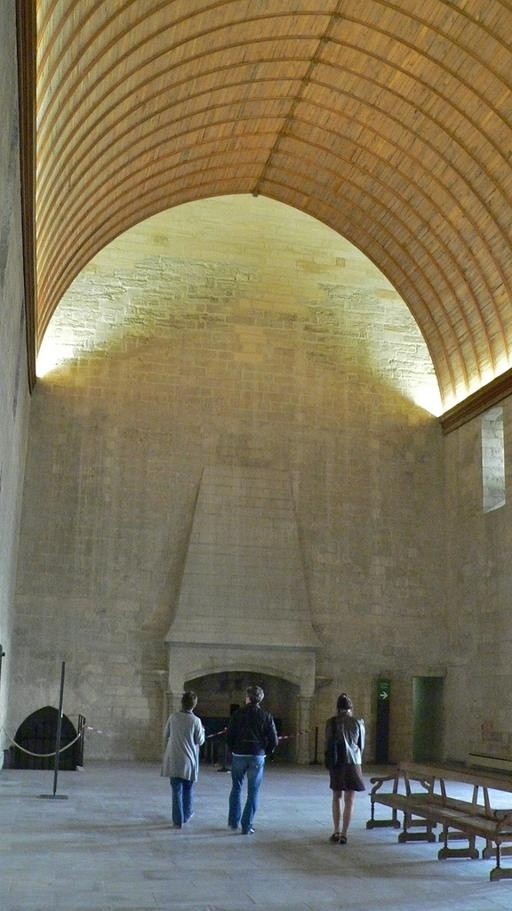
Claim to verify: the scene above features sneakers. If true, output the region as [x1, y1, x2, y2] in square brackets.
[217, 766, 231, 773]
[245, 827, 256, 834]
[331, 832, 347, 844]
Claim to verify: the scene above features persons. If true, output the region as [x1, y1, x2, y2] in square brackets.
[217, 702, 242, 776]
[154, 688, 208, 829]
[323, 691, 367, 844]
[225, 683, 281, 836]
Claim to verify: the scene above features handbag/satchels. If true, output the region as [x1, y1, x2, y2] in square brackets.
[324, 744, 338, 771]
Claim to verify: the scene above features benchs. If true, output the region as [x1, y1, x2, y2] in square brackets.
[366, 762, 512, 882]
